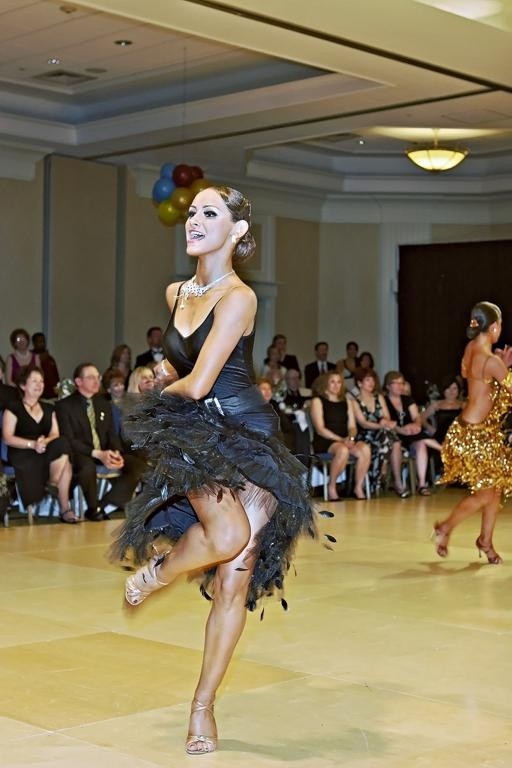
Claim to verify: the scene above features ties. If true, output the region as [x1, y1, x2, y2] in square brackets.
[87, 398, 101, 450]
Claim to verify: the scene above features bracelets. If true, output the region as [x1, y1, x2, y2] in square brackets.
[156, 356, 173, 383]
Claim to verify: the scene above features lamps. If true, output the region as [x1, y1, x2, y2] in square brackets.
[404, 129, 471, 173]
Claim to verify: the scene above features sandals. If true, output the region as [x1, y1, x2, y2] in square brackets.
[125, 550, 177, 605]
[395, 488, 411, 498]
[416, 486, 430, 496]
[58, 509, 81, 523]
[184, 697, 219, 756]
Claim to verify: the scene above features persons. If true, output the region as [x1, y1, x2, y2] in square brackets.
[256, 334, 466, 502]
[3, 365, 83, 524]
[118, 179, 317, 758]
[1, 327, 61, 409]
[102, 326, 181, 511]
[430, 298, 512, 568]
[53, 362, 139, 522]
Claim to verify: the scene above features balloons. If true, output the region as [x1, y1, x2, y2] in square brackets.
[152, 156, 212, 226]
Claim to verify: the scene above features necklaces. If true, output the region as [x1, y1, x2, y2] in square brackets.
[173, 269, 239, 310]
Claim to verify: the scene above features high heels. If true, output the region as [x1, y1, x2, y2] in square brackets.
[428, 521, 451, 558]
[475, 534, 504, 565]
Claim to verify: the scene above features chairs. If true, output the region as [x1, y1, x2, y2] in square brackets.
[2, 411, 443, 523]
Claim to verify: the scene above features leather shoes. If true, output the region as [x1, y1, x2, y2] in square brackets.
[85, 508, 107, 520]
